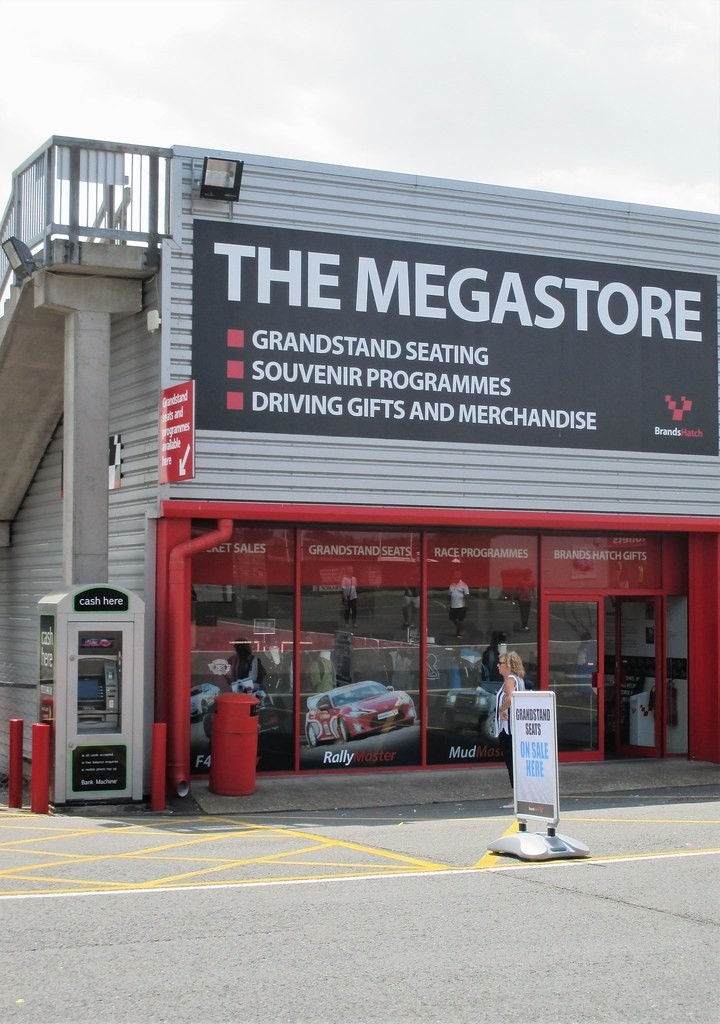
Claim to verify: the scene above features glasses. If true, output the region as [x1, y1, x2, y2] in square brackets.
[498, 661, 506, 665]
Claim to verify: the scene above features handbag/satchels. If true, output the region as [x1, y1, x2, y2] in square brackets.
[342, 594, 351, 606]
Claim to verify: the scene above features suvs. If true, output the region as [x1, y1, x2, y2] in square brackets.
[442, 680, 535, 739]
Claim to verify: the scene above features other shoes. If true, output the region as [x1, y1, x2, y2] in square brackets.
[352, 623, 358, 628]
[344, 624, 349, 628]
[401, 622, 409, 629]
[500, 802, 515, 808]
[410, 623, 415, 629]
[456, 632, 463, 638]
[522, 625, 529, 632]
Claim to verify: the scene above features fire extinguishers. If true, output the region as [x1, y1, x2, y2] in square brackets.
[648, 678, 678, 727]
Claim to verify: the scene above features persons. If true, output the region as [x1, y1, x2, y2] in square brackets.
[401, 562, 419, 630]
[248, 642, 336, 693]
[483, 631, 506, 682]
[447, 570, 470, 638]
[224, 638, 266, 691]
[515, 569, 534, 632]
[342, 565, 377, 628]
[495, 652, 525, 809]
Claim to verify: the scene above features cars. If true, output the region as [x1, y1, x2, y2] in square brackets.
[306, 680, 416, 746]
[189, 683, 220, 719]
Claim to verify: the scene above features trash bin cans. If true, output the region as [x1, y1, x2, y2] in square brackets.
[208, 692, 260, 797]
